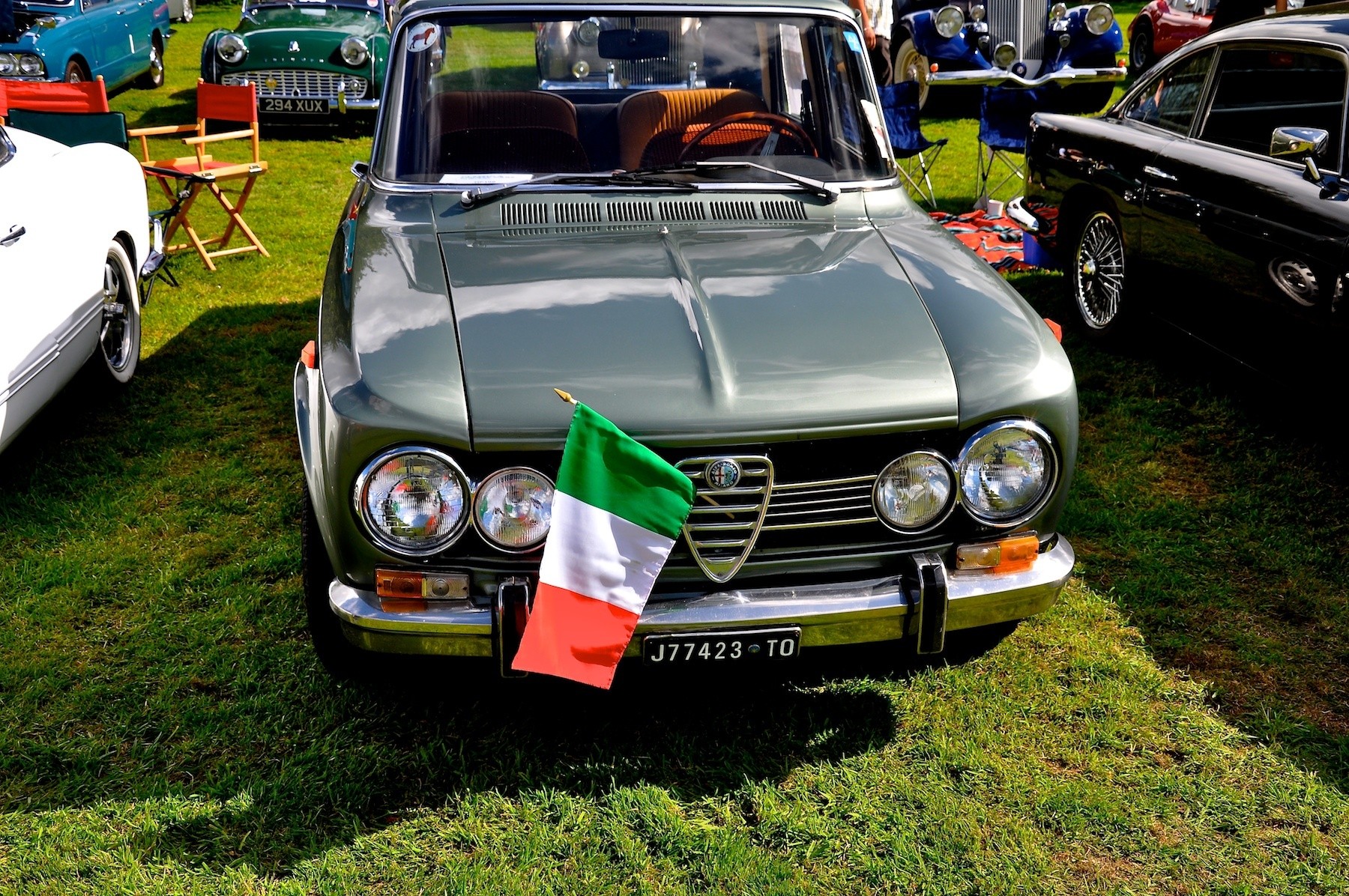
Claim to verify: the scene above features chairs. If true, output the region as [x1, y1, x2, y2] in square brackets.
[425, 89, 580, 165]
[7, 106, 187, 309]
[0, 75, 112, 128]
[973, 82, 1049, 208]
[127, 77, 272, 272]
[876, 78, 950, 210]
[616, 84, 769, 169]
[428, 126, 592, 173]
[637, 120, 805, 167]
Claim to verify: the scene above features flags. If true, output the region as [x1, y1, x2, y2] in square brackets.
[513, 398, 696, 693]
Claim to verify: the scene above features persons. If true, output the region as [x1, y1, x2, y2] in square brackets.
[1027, 122, 1056, 190]
[1020, 466, 1034, 489]
[848, 0, 895, 89]
[1128, 78, 1167, 126]
[1208, 0, 1288, 38]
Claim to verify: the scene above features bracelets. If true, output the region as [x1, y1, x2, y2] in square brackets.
[863, 26, 871, 31]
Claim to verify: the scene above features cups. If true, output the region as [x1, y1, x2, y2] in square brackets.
[987, 198, 1005, 216]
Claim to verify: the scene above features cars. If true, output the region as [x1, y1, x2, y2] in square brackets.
[201, 1, 392, 137]
[1, 125, 150, 454]
[292, 0, 1082, 669]
[0, 0, 170, 89]
[1023, 15, 1348, 414]
